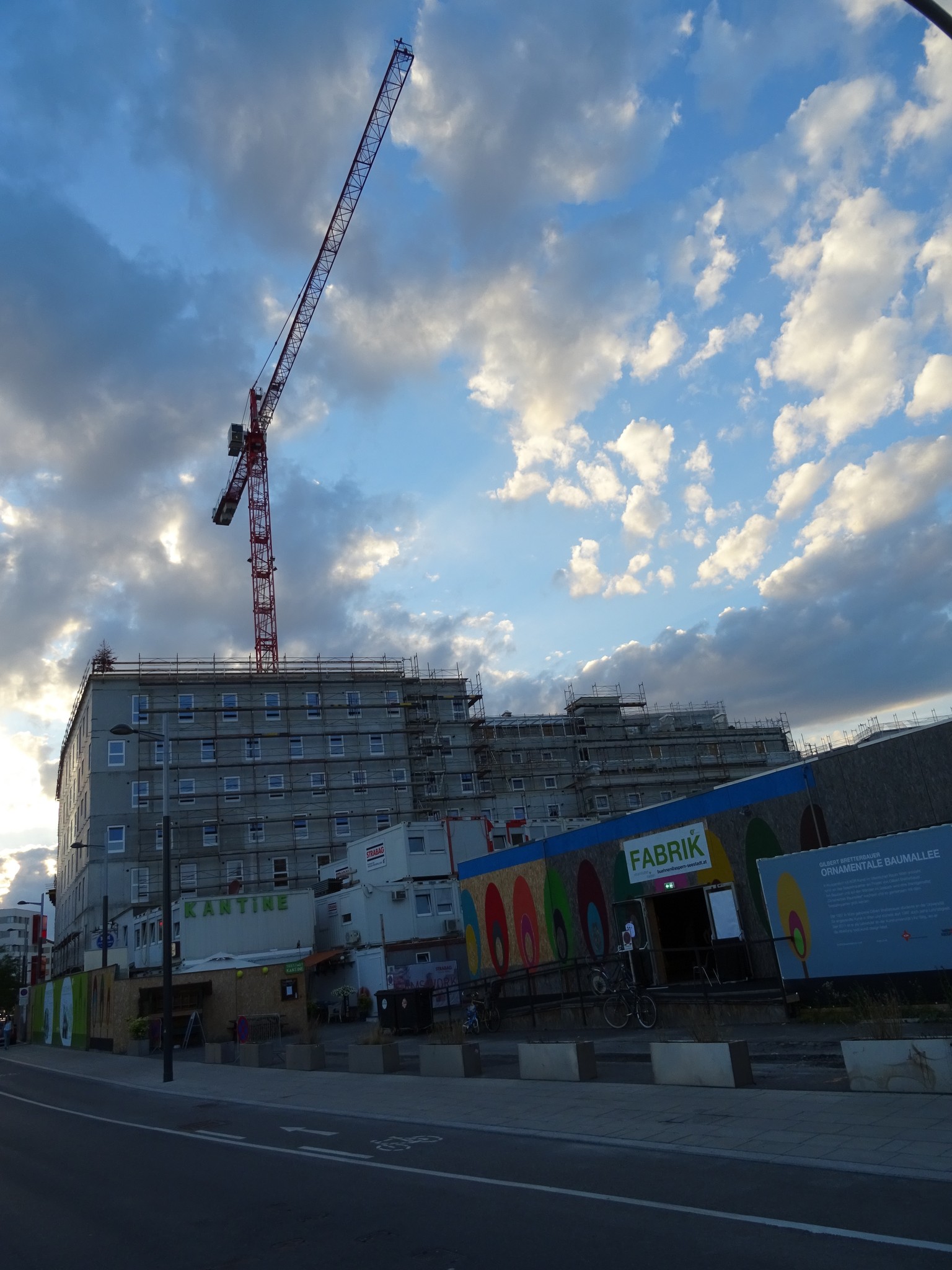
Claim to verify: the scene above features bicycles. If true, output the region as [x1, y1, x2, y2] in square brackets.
[586, 947, 637, 996]
[461, 991, 500, 1033]
[603, 978, 657, 1029]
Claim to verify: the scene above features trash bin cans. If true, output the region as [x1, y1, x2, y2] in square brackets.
[374, 985, 437, 1037]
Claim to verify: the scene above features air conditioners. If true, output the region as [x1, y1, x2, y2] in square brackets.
[345, 930, 359, 944]
[444, 919, 460, 932]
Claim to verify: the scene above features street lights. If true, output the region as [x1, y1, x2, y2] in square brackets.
[108, 713, 172, 1080]
[6, 918, 28, 985]
[70, 830, 108, 967]
[17, 893, 44, 984]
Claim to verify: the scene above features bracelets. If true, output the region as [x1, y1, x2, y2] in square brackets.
[12, 1030, 14, 1030]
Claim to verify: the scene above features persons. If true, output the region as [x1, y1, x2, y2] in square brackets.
[1, 1015, 14, 1051]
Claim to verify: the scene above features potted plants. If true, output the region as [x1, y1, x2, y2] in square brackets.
[124, 986, 952, 1094]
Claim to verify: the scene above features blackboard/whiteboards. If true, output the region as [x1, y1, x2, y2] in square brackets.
[185, 1011, 205, 1044]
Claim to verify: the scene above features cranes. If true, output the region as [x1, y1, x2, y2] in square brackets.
[212, 36, 418, 673]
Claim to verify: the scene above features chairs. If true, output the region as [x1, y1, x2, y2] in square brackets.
[328, 1000, 344, 1024]
